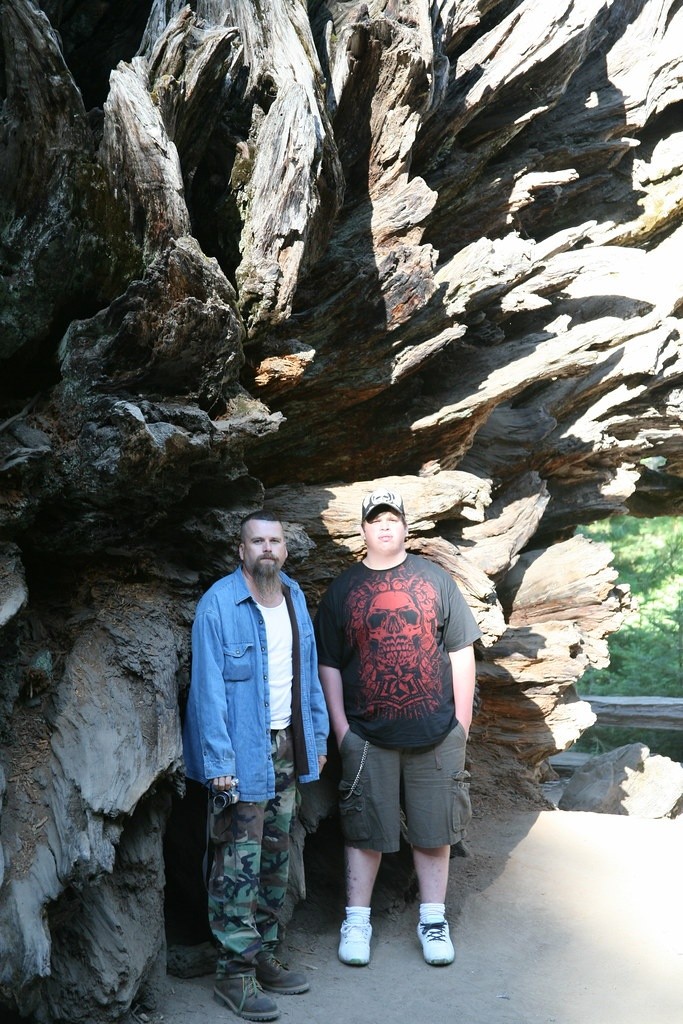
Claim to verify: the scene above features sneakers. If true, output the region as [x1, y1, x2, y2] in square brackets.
[417, 920, 456, 965]
[337, 920, 373, 967]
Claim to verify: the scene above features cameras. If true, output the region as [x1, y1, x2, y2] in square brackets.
[213, 778, 240, 809]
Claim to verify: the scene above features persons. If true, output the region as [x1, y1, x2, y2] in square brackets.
[314, 488, 482, 965]
[184, 510, 329, 1021]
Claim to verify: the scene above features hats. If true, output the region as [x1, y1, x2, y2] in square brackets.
[360, 491, 405, 524]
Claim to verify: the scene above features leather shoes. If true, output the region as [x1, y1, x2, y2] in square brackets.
[214, 975, 280, 1021]
[256, 947, 309, 995]
